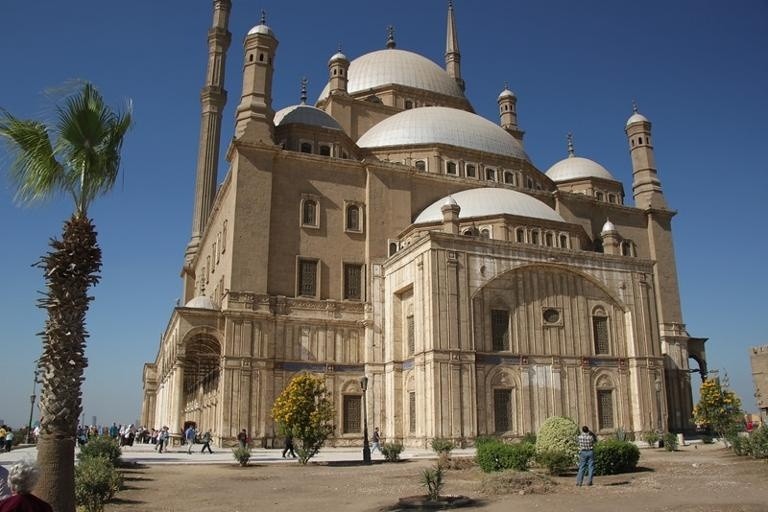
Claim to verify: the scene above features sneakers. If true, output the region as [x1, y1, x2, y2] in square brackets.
[120, 442, 215, 454]
[283, 455, 297, 459]
[576, 482, 593, 486]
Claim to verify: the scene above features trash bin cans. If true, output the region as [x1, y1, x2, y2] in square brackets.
[677, 433, 684, 446]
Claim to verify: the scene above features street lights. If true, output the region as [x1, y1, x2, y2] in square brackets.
[359, 377, 373, 463]
[25, 393, 37, 444]
[81, 412, 85, 427]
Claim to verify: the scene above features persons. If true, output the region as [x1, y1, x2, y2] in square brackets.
[371, 427, 382, 454]
[0, 460, 53, 512]
[0, 422, 253, 454]
[575, 426, 595, 486]
[0, 465, 11, 499]
[282, 432, 296, 458]
